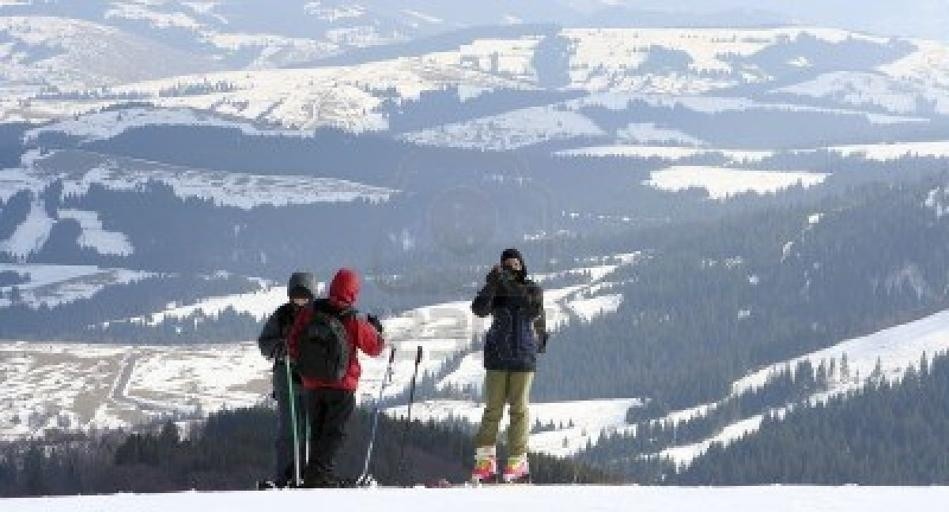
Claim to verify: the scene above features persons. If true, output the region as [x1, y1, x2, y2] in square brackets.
[466, 247, 549, 483]
[287, 266, 385, 488]
[254, 272, 320, 488]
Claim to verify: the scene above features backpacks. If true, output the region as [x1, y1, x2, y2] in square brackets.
[294, 299, 361, 384]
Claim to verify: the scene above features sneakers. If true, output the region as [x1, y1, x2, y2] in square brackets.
[499, 453, 531, 482]
[470, 455, 497, 481]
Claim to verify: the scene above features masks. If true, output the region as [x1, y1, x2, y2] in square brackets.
[293, 298, 310, 307]
